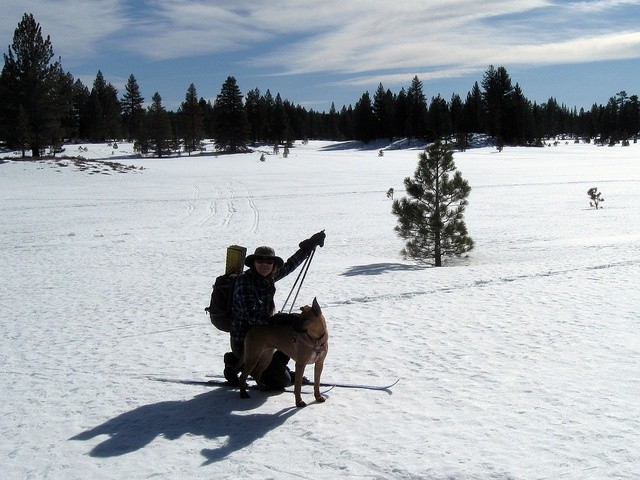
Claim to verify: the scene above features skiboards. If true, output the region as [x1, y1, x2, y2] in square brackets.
[147, 375, 398, 396]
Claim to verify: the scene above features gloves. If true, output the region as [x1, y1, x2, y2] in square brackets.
[298, 231, 325, 253]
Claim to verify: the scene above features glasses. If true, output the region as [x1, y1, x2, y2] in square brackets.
[256, 257, 274, 264]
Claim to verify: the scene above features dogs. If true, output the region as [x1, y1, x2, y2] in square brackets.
[239, 296, 329, 408]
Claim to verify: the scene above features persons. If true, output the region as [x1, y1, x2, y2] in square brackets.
[223, 231, 328, 388]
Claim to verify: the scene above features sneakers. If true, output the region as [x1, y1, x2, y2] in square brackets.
[290, 371, 309, 383]
[224, 352, 240, 381]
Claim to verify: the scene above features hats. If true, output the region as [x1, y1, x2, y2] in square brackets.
[244, 246, 285, 269]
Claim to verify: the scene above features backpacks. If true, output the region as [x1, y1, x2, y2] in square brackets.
[205, 272, 239, 332]
